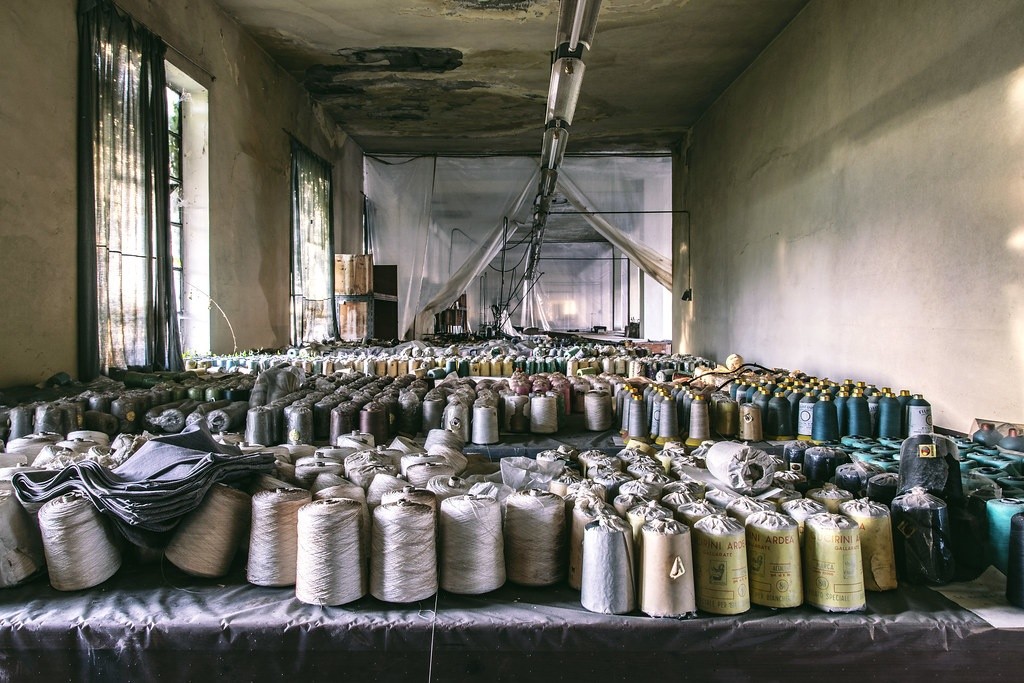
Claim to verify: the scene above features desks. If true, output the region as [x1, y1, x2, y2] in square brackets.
[0, 558, 1024, 683]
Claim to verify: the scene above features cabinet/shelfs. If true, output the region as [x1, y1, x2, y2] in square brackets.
[434, 293, 467, 333]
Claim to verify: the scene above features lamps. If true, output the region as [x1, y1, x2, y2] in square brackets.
[538, 126, 568, 169]
[544, 54, 587, 126]
[525, 166, 559, 280]
[554, 0, 603, 52]
